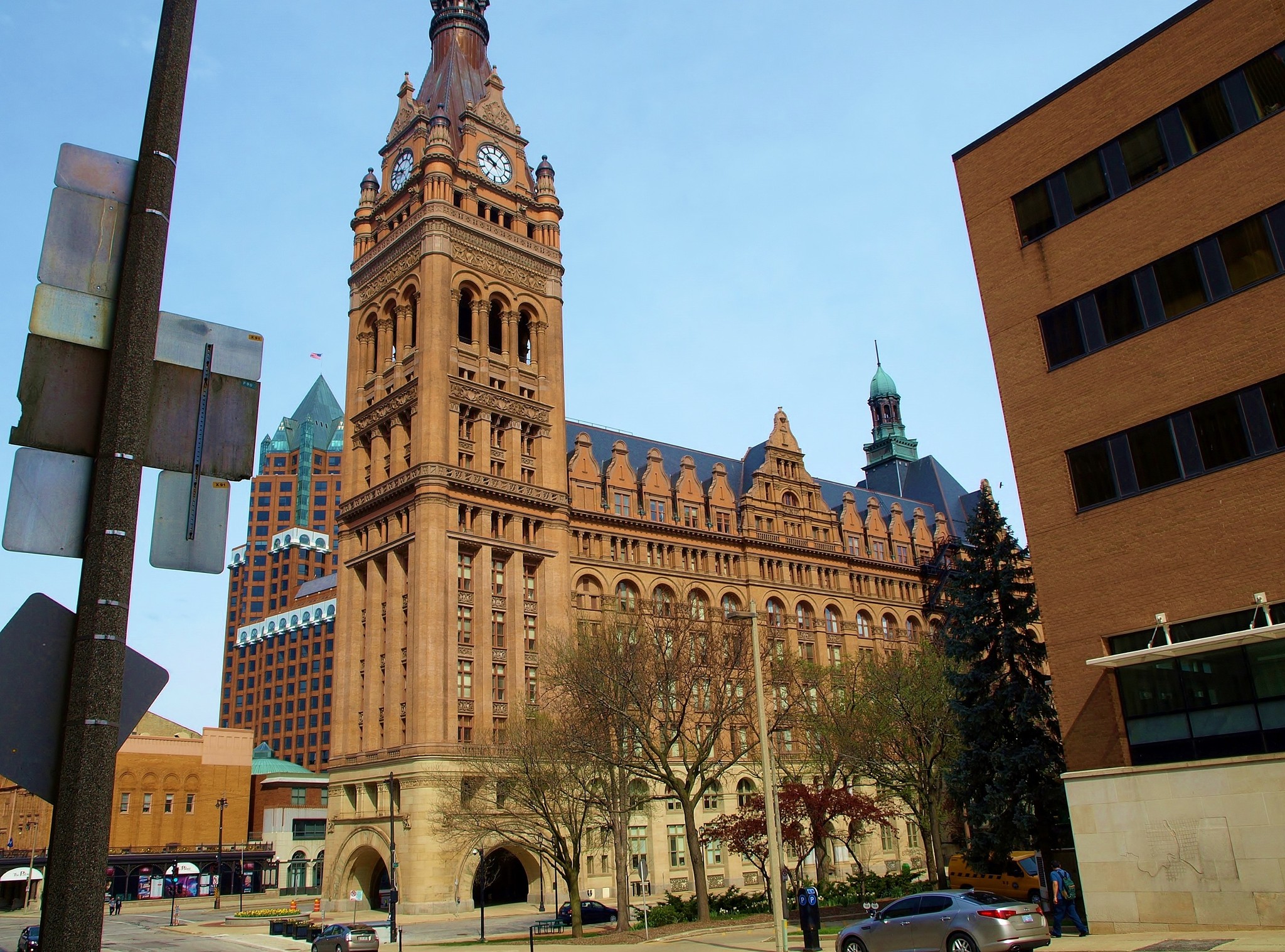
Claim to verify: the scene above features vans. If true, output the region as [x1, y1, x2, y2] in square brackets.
[948, 850, 1042, 906]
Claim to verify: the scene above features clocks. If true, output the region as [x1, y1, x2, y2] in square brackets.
[390, 150, 414, 192]
[476, 143, 513, 185]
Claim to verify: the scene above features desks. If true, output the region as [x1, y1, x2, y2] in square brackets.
[535, 919, 564, 933]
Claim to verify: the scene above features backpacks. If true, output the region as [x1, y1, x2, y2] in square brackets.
[1055, 870, 1076, 900]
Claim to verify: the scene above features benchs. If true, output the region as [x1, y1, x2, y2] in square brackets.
[532, 924, 568, 935]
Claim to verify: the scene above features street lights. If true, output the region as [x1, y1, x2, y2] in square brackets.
[550, 834, 561, 928]
[725, 600, 785, 952]
[537, 832, 547, 912]
[383, 771, 398, 943]
[473, 847, 488, 944]
[276, 856, 280, 888]
[23, 820, 38, 914]
[213, 798, 229, 896]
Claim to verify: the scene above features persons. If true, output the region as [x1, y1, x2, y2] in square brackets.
[242, 875, 252, 893]
[115, 896, 122, 915]
[1046, 860, 1090, 939]
[108, 897, 116, 916]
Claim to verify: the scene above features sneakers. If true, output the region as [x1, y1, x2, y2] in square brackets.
[1049, 930, 1061, 938]
[1079, 931, 1088, 937]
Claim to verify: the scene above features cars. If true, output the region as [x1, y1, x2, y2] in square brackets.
[835, 888, 1052, 952]
[310, 924, 379, 952]
[559, 900, 618, 926]
[17, 925, 40, 952]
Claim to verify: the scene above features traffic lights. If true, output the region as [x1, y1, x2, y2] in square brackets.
[174, 867, 179, 883]
[171, 865, 174, 882]
[178, 883, 183, 894]
